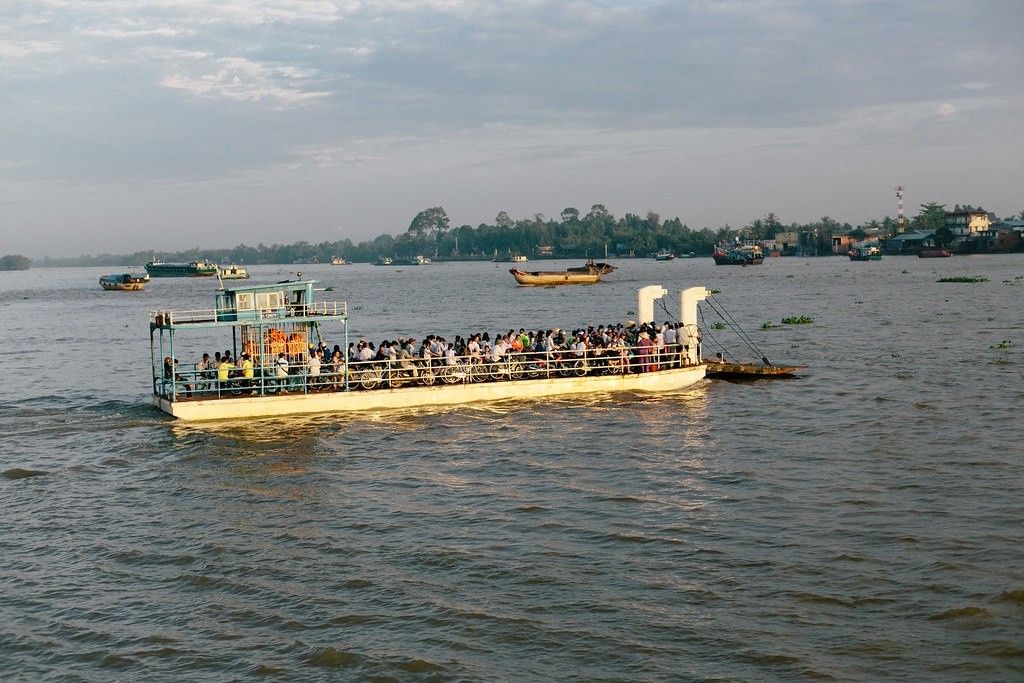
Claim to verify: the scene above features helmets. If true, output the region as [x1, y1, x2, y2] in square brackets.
[554, 328, 561, 334]
[242, 351, 246, 356]
[164, 357, 171, 363]
[321, 342, 327, 347]
[360, 339, 366, 343]
[308, 343, 314, 349]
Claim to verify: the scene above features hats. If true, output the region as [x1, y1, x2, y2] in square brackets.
[398, 337, 403, 341]
[639, 332, 650, 339]
[278, 353, 285, 356]
[503, 335, 508, 340]
[409, 338, 417, 341]
[650, 321, 658, 325]
[626, 320, 635, 328]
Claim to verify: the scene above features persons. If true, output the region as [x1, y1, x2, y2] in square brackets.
[164, 357, 194, 400]
[198, 321, 703, 399]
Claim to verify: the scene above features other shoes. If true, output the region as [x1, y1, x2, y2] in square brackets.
[285, 390, 288, 393]
[309, 390, 311, 392]
[187, 397, 194, 400]
[316, 390, 319, 392]
[221, 396, 223, 398]
[627, 370, 634, 374]
[279, 392, 281, 395]
[176, 393, 181, 396]
[250, 392, 253, 395]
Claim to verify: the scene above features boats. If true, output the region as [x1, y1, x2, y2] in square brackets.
[713, 245, 765, 265]
[144, 260, 215, 277]
[99, 271, 148, 290]
[147, 283, 808, 423]
[656, 252, 673, 261]
[918, 250, 950, 259]
[848, 246, 882, 261]
[510, 262, 618, 286]
[680, 252, 694, 258]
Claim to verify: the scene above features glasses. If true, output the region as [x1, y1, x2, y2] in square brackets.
[520, 332, 523, 334]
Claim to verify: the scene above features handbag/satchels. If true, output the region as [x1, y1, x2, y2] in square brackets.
[650, 363, 657, 372]
